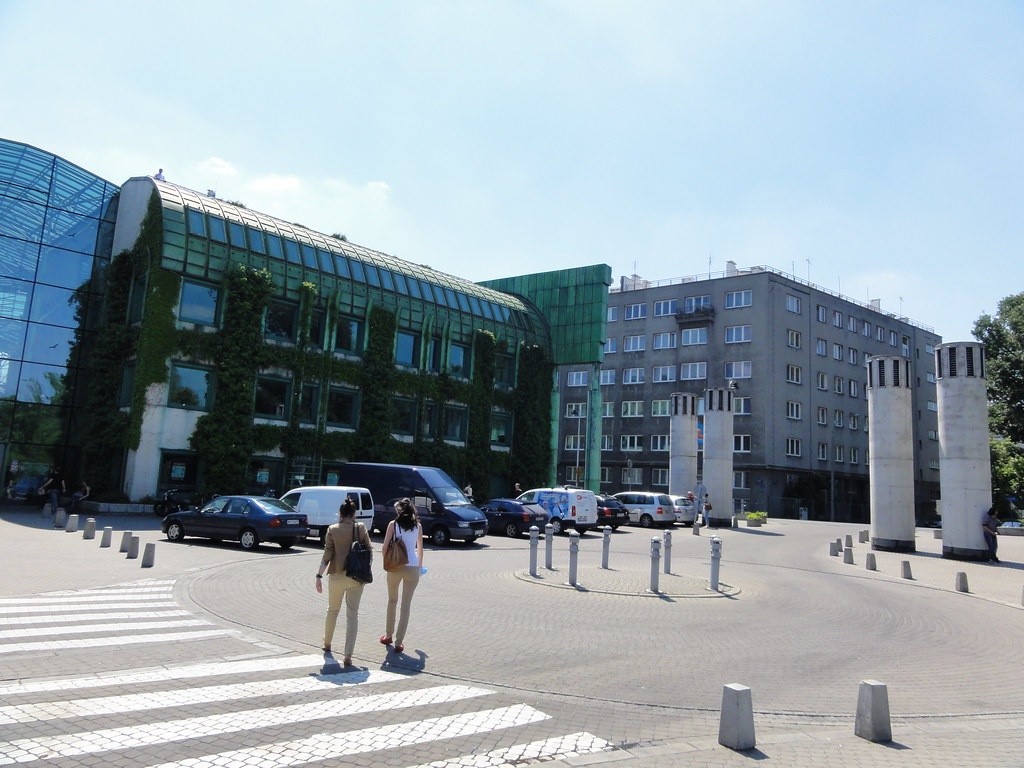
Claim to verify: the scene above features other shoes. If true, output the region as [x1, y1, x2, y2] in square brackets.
[380, 635, 393, 644]
[395, 644, 404, 653]
[322, 645, 331, 651]
[343, 658, 353, 665]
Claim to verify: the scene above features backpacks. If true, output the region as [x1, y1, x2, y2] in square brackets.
[463, 489, 468, 494]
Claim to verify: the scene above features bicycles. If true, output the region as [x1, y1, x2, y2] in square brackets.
[154, 489, 187, 517]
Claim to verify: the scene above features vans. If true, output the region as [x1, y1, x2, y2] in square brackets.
[612, 491, 676, 529]
[514, 488, 598, 536]
[337, 462, 489, 547]
[279, 486, 374, 547]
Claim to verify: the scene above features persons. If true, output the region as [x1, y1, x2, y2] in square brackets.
[463, 482, 472, 504]
[702, 492, 712, 529]
[982, 507, 1003, 563]
[687, 491, 695, 503]
[513, 483, 522, 497]
[316, 497, 373, 665]
[380, 497, 423, 651]
[264, 490, 275, 498]
[68, 480, 92, 514]
[37, 467, 66, 514]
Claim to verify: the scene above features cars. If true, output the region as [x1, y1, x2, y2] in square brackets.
[478, 499, 549, 538]
[588, 494, 630, 531]
[14, 475, 47, 501]
[160, 495, 311, 551]
[667, 495, 699, 526]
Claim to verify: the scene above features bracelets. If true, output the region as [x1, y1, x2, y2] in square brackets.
[42, 485, 45, 488]
[62, 488, 66, 490]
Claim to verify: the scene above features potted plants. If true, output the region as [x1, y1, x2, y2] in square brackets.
[746, 512, 761, 527]
[756, 511, 768, 524]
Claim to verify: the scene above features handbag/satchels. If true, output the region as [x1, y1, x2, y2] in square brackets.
[38, 489, 45, 495]
[383, 521, 409, 572]
[344, 523, 373, 584]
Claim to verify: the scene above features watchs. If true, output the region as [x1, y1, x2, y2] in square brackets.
[316, 573, 323, 578]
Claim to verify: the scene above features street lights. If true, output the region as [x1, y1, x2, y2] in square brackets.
[571, 410, 581, 487]
